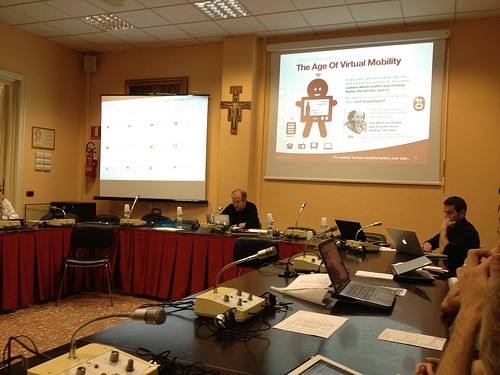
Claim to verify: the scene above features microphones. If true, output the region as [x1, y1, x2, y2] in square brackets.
[284, 202, 309, 238]
[119, 194, 146, 227]
[195, 226, 338, 321]
[47, 206, 75, 226]
[27, 307, 167, 375]
[355, 221, 382, 241]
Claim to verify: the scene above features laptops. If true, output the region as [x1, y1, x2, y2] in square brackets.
[317, 238, 396, 310]
[283, 355, 365, 375]
[392, 256, 435, 283]
[386, 227, 448, 258]
[335, 220, 390, 247]
[206, 215, 230, 226]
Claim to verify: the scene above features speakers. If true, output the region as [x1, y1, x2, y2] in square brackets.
[84, 55, 97, 72]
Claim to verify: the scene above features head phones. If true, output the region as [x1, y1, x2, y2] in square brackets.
[259, 292, 278, 315]
[215, 311, 241, 333]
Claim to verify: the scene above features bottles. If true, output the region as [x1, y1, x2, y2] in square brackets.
[267, 221, 275, 239]
[124, 210, 130, 219]
[177, 214, 183, 229]
[321, 224, 327, 238]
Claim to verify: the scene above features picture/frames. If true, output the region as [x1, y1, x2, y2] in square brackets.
[32, 126, 55, 150]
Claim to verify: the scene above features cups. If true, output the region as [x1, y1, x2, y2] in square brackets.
[321, 217, 327, 226]
[267, 213, 273, 222]
[177, 206, 183, 215]
[124, 204, 130, 212]
[307, 230, 313, 244]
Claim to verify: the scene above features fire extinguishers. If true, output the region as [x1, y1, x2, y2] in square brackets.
[86, 141, 97, 177]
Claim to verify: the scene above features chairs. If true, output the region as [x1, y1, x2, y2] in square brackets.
[54, 222, 116, 308]
[365, 232, 386, 243]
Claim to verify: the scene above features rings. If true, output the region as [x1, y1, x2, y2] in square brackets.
[463, 264, 467, 267]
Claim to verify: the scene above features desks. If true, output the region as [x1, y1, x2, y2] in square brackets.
[0, 219, 440, 375]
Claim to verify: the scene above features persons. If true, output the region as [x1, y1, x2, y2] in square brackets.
[415, 188, 500, 375]
[346, 110, 365, 134]
[0, 186, 19, 221]
[422, 196, 479, 266]
[225, 98, 245, 128]
[221, 189, 260, 229]
